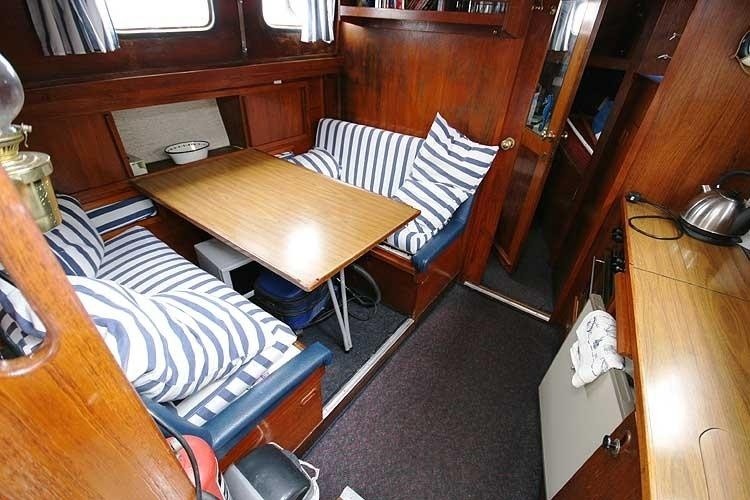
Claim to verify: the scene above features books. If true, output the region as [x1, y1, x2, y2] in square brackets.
[375, 0, 454, 12]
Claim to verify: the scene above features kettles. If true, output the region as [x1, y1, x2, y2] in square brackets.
[678, 169, 750, 246]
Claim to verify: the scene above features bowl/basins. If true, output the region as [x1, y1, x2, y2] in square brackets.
[163, 140, 209, 165]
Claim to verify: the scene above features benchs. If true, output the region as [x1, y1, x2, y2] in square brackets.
[95, 224, 334, 477]
[278, 117, 474, 321]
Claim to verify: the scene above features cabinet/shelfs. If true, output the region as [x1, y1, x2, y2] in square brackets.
[493, 3, 749, 325]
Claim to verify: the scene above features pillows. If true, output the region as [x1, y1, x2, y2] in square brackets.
[286, 146, 343, 180]
[64, 274, 277, 402]
[44, 192, 104, 279]
[380, 114, 499, 254]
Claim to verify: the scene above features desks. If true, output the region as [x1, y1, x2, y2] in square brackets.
[133, 146, 420, 352]
[549, 264, 750, 500]
[555, 195, 746, 298]
